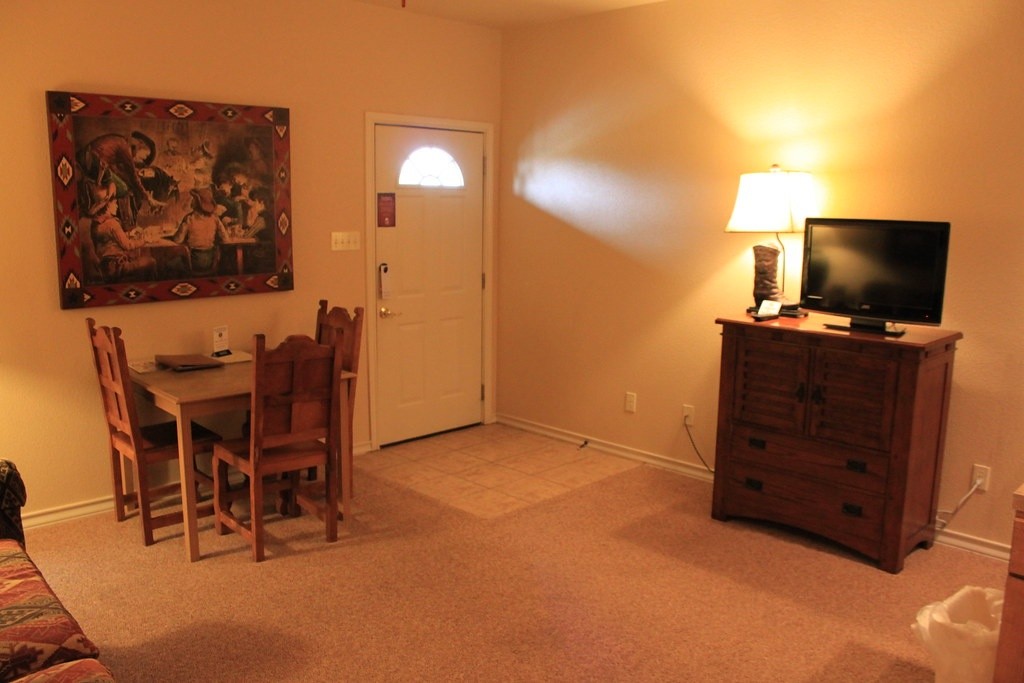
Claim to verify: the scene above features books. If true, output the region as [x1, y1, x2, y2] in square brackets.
[155, 353, 225, 371]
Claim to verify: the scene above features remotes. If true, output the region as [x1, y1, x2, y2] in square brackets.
[752, 313, 779, 322]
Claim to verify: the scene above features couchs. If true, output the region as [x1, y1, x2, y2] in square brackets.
[0, 457, 119, 683]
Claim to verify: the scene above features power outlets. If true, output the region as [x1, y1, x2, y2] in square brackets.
[624, 392, 636, 413]
[682, 404, 695, 427]
[969, 464, 989, 493]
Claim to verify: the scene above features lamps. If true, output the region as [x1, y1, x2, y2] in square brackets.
[723, 164, 832, 318]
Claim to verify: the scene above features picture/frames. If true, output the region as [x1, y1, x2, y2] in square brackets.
[44, 90, 295, 311]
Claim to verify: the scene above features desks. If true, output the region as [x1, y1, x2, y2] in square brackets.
[126, 349, 360, 563]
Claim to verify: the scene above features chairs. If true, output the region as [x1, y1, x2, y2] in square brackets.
[218, 299, 364, 562]
[84, 316, 232, 546]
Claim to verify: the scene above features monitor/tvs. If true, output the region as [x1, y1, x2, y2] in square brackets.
[799, 218, 951, 336]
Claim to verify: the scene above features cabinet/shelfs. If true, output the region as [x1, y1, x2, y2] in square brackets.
[711, 308, 964, 574]
